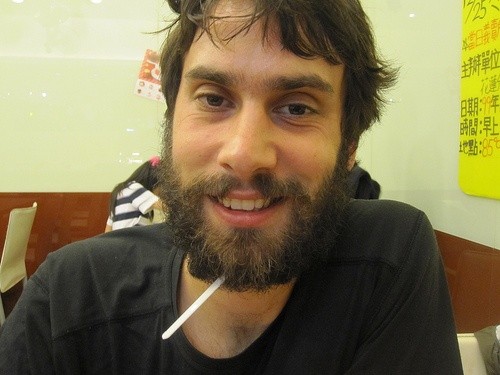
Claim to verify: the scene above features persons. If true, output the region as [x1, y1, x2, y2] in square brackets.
[347, 162, 378, 200]
[105, 156, 166, 232]
[0, 0, 463, 375]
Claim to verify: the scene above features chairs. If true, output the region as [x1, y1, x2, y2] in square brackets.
[0, 201, 38, 327]
